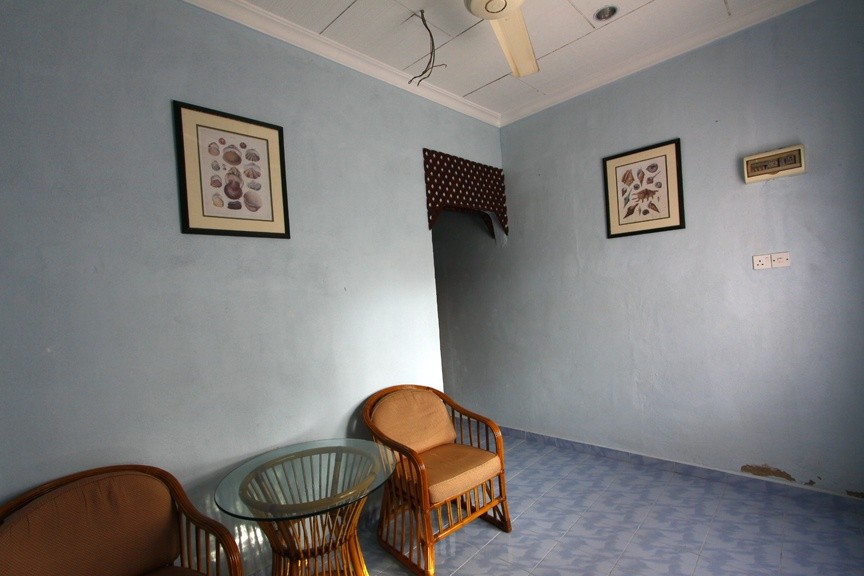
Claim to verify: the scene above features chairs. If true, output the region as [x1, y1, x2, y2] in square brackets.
[0, 464, 244, 576]
[362, 383, 512, 576]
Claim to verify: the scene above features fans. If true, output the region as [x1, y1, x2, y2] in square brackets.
[464, 0, 540, 78]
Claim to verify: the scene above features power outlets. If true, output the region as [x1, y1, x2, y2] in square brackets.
[752, 251, 792, 270]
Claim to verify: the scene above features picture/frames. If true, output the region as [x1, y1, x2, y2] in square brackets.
[601, 136, 686, 240]
[169, 99, 291, 239]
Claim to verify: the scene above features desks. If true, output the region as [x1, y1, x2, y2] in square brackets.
[213, 438, 398, 576]
